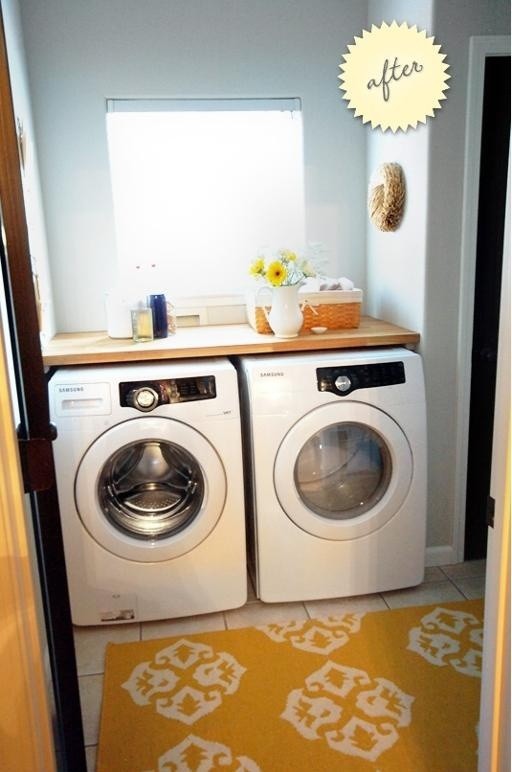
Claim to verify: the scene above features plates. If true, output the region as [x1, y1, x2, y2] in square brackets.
[310, 326, 327, 333]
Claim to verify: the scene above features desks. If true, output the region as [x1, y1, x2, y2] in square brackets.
[38, 311, 424, 369]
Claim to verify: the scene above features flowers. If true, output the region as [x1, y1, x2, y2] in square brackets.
[248, 249, 318, 289]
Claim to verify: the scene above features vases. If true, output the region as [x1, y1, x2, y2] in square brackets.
[255, 281, 310, 342]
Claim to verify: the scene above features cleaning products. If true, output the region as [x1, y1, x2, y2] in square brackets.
[131, 300, 153, 344]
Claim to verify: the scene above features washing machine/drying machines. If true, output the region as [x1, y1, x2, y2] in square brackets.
[46, 356, 249, 630]
[233, 346, 429, 605]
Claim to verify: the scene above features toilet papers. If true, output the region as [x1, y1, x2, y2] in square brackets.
[105, 294, 136, 340]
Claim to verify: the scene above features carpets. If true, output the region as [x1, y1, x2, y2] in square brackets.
[87, 592, 490, 772]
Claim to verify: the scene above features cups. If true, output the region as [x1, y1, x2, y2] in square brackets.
[105, 296, 148, 339]
[132, 310, 154, 342]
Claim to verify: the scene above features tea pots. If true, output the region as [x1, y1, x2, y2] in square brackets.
[257, 281, 307, 338]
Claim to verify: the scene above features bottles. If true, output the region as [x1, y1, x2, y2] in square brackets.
[150, 294, 168, 340]
